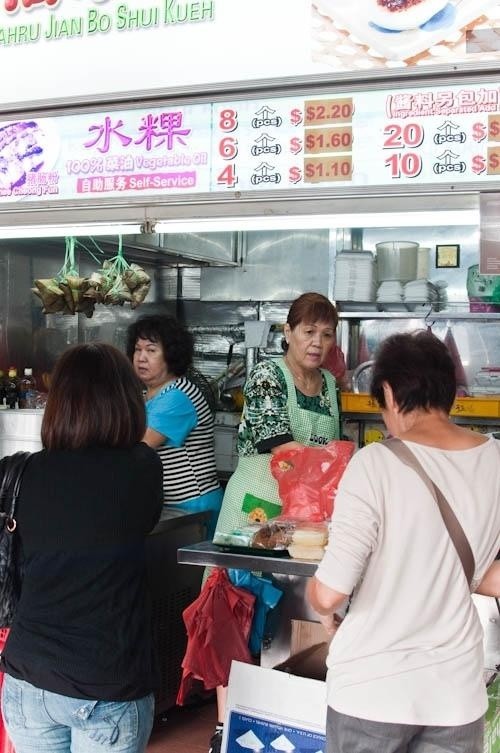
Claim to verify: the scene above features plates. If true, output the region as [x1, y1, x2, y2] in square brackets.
[351, 359, 376, 394]
[375, 280, 440, 303]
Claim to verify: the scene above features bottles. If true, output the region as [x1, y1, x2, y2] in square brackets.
[0, 366, 37, 410]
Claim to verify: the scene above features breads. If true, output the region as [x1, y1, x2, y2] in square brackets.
[212, 518, 328, 560]
[366, 0, 449, 31]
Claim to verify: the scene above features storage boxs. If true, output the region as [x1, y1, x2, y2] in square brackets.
[220, 617, 340, 753]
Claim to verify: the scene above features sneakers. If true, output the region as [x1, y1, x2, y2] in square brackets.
[208, 733, 222, 753]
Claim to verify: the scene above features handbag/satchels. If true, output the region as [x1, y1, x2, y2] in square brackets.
[0, 450, 39, 631]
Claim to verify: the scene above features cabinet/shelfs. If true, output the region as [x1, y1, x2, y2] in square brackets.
[63, 231, 247, 269]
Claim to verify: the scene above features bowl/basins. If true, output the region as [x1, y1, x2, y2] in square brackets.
[376, 241, 419, 281]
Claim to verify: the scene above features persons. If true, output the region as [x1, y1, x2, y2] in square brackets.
[1, 344, 170, 752]
[20, 351, 58, 393]
[305, 325, 500, 752]
[120, 314, 223, 550]
[201, 292, 339, 753]
